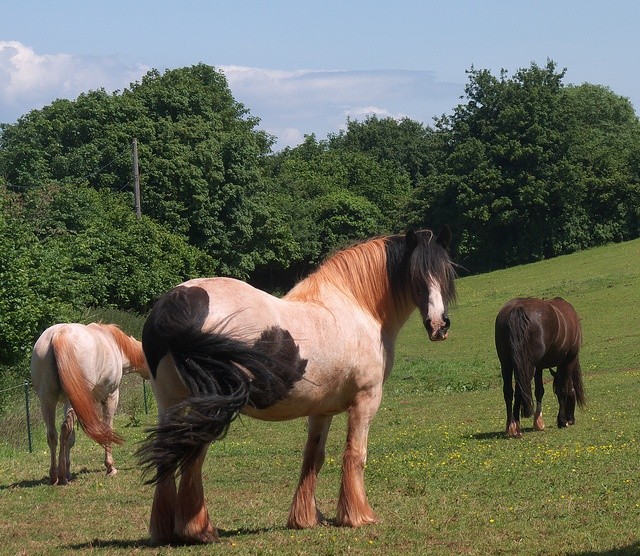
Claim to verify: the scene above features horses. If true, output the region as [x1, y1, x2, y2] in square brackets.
[132, 229, 469, 543]
[497, 297, 588, 438]
[30, 322, 148, 487]
